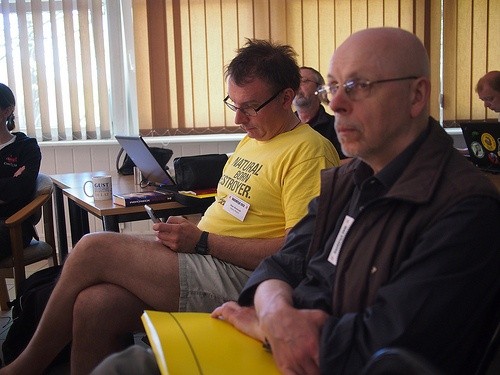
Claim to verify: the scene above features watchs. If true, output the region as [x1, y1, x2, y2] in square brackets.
[196, 232, 209, 255]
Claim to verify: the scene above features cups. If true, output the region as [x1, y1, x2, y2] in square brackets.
[133, 166, 143, 185]
[83, 176, 112, 201]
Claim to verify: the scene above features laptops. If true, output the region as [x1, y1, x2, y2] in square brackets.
[460, 121, 500, 174]
[114, 136, 212, 192]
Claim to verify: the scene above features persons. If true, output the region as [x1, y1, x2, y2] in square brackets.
[476, 70, 500, 122]
[293, 68, 353, 165]
[0, 37, 340, 375]
[92, 27, 500, 375]
[0, 83, 42, 260]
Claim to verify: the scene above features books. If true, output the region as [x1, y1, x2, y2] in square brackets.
[114, 192, 164, 206]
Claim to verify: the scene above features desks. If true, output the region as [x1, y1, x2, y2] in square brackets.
[62, 183, 200, 248]
[43, 171, 135, 264]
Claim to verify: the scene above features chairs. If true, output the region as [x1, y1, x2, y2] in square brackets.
[0, 172, 59, 312]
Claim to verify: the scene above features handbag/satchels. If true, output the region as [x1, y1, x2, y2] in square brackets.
[2, 265, 135, 375]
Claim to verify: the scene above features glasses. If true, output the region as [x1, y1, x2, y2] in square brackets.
[314, 76, 417, 105]
[223, 92, 279, 117]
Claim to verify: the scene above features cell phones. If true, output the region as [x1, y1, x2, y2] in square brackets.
[144, 204, 162, 223]
